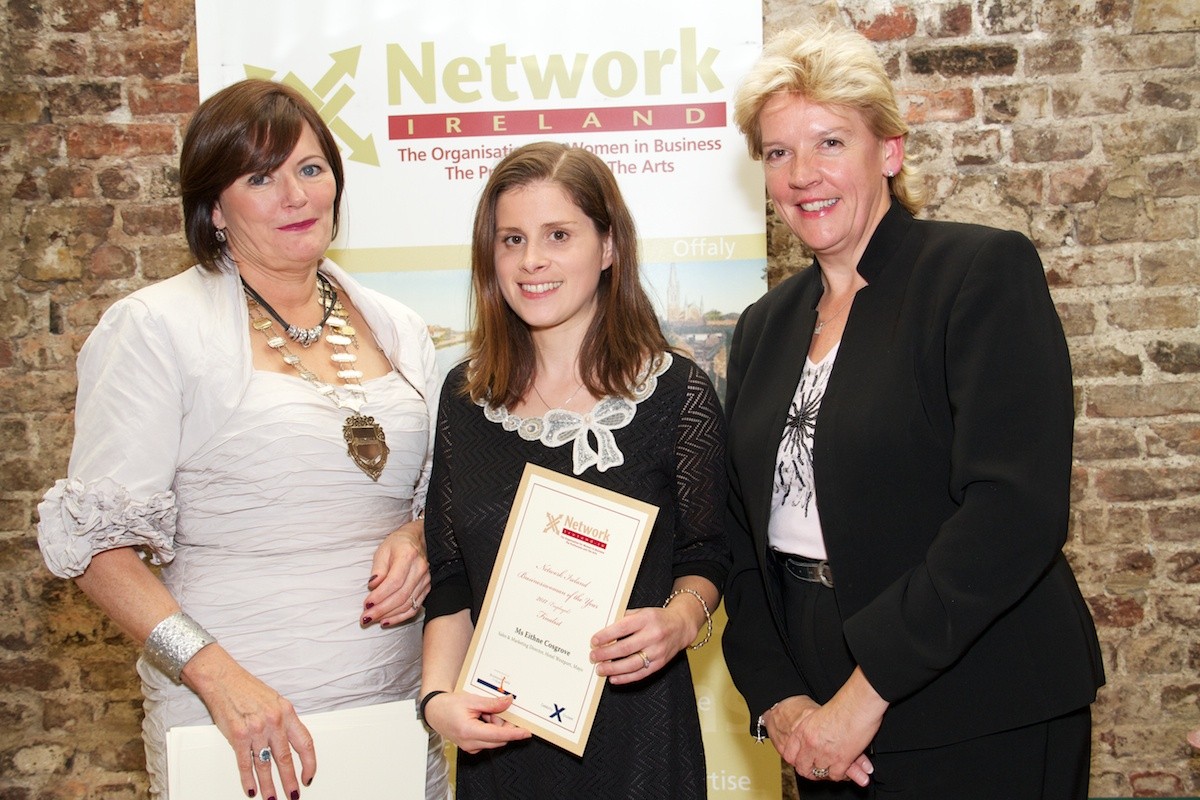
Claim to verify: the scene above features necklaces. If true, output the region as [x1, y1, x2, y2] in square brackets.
[241, 272, 392, 482]
[813, 293, 855, 335]
[533, 381, 584, 409]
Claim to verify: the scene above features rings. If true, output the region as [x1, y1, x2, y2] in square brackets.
[639, 651, 649, 668]
[812, 767, 828, 777]
[254, 747, 271, 761]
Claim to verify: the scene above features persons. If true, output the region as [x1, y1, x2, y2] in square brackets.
[723, 23, 1106, 800]
[422, 143, 725, 799]
[37, 80, 439, 800]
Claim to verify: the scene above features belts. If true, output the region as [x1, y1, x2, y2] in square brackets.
[781, 553, 835, 588]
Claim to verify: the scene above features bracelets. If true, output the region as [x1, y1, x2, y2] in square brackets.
[143, 612, 214, 685]
[663, 589, 711, 650]
[753, 702, 778, 744]
[421, 691, 446, 730]
